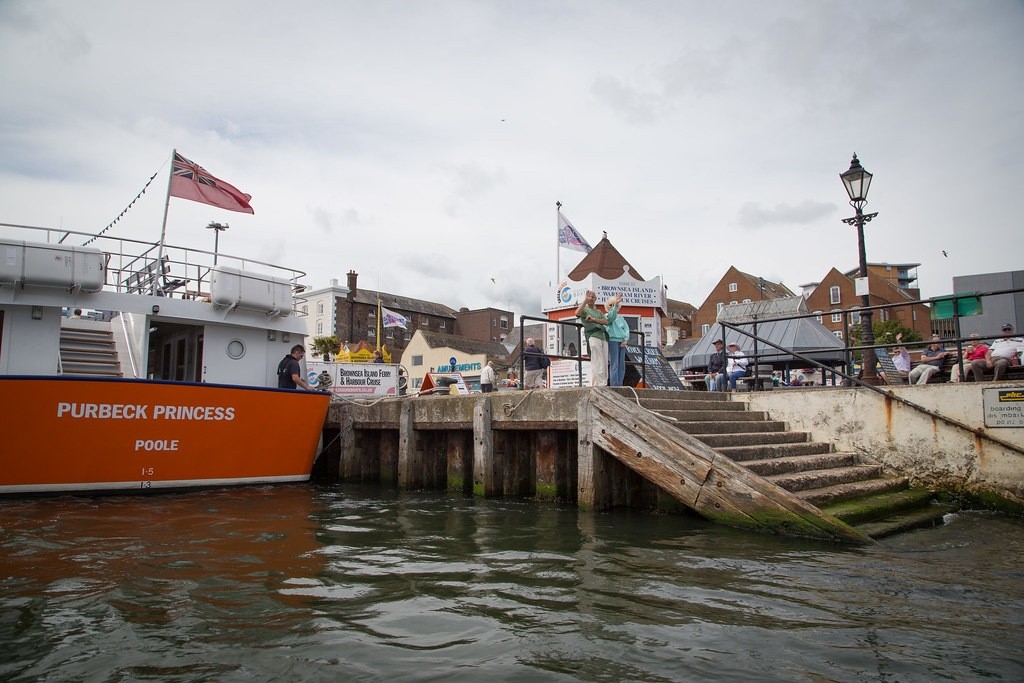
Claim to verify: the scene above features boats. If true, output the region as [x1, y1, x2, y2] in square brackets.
[0, 149, 333, 492]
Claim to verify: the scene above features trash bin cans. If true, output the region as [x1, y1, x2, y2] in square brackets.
[752, 365, 774, 391]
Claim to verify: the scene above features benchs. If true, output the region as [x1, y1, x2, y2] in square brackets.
[903, 356, 1024, 384]
[126, 255, 190, 298]
[685, 374, 773, 392]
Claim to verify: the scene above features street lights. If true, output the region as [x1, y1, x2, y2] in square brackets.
[839, 152, 883, 386]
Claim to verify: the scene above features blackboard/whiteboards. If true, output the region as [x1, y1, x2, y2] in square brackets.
[625, 344, 687, 390]
[855, 348, 905, 385]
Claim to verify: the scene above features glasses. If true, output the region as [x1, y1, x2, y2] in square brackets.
[729, 345, 734, 347]
[298, 351, 303, 357]
[895, 351, 900, 353]
[1003, 328, 1011, 331]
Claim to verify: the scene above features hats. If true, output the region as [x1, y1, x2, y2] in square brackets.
[1002, 324, 1013, 331]
[712, 339, 723, 344]
[727, 343, 741, 351]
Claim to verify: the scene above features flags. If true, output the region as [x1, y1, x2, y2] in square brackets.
[168, 152, 255, 214]
[557, 212, 593, 253]
[380, 306, 408, 329]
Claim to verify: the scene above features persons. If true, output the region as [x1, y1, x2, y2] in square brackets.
[372, 351, 384, 363]
[877, 333, 948, 384]
[68, 308, 83, 320]
[774, 372, 804, 387]
[704, 339, 748, 393]
[574, 290, 631, 388]
[481, 361, 495, 393]
[276, 344, 310, 390]
[947, 324, 1024, 383]
[523, 336, 550, 390]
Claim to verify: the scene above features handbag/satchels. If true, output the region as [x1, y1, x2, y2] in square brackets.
[538, 348, 551, 368]
[1010, 352, 1020, 365]
[745, 370, 751, 376]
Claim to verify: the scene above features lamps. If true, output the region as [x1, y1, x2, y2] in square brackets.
[31, 306, 43, 319]
[152, 305, 159, 314]
